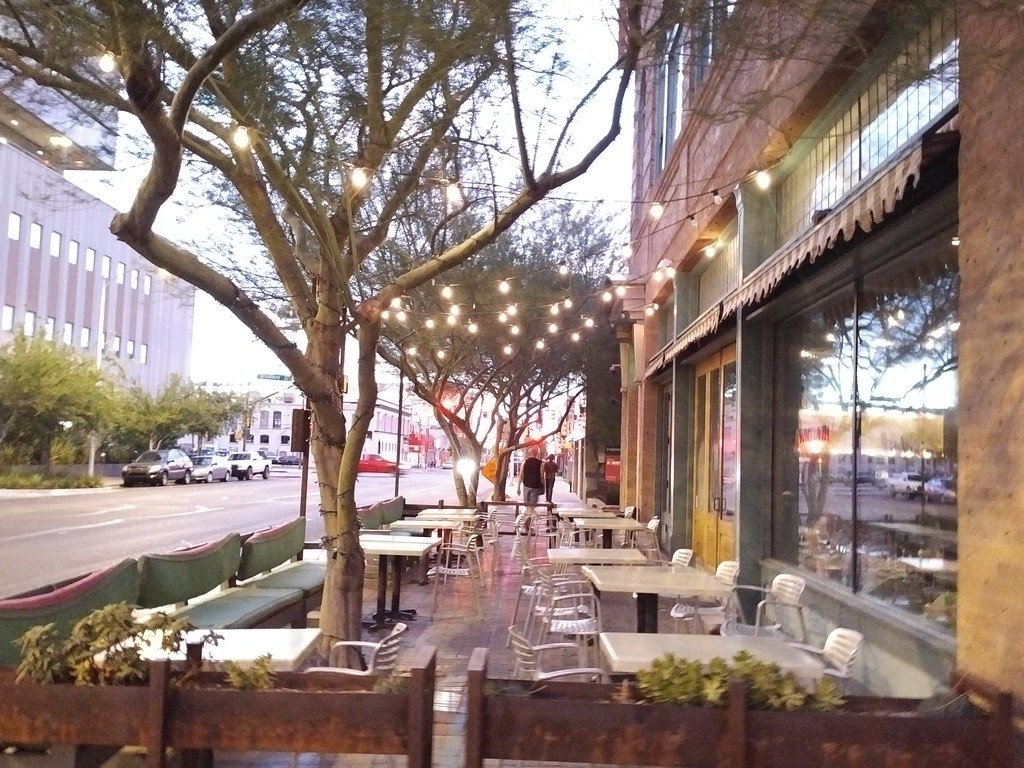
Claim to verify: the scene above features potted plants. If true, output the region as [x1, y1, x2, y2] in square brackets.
[1, 602, 1017, 768]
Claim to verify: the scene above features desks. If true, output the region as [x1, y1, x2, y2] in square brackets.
[581, 563, 733, 703]
[358, 541, 433, 632]
[872, 520, 957, 580]
[545, 547, 648, 646]
[92, 626, 322, 672]
[573, 517, 644, 567]
[359, 534, 443, 621]
[388, 508, 481, 587]
[555, 506, 615, 546]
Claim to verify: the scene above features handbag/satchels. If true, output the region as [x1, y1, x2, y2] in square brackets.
[539, 484, 545, 495]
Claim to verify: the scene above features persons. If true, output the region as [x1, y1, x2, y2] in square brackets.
[517, 446, 544, 532]
[543, 454, 558, 504]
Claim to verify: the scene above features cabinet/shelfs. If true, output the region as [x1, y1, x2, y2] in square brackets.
[598, 630, 827, 678]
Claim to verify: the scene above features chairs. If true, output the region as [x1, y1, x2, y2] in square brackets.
[293, 623, 409, 768]
[426, 506, 604, 684]
[800, 515, 871, 586]
[721, 572, 807, 643]
[616, 504, 744, 634]
[787, 626, 866, 696]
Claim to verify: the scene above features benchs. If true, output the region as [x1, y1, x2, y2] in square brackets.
[357, 496, 422, 585]
[0, 516, 327, 675]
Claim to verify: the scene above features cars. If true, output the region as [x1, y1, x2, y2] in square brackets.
[357, 454, 402, 474]
[886, 472, 957, 504]
[829, 466, 876, 486]
[122, 447, 302, 489]
[441, 461, 453, 470]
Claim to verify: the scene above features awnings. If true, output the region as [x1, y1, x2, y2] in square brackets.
[644, 130, 961, 378]
